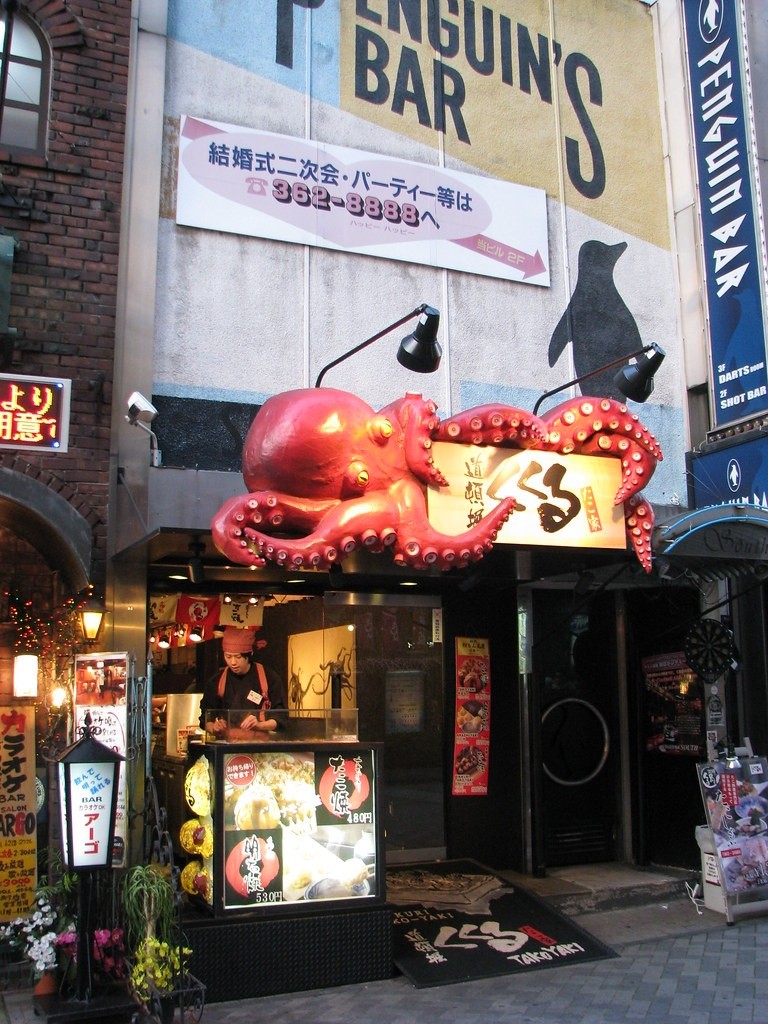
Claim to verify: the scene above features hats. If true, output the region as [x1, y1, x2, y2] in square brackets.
[222, 627, 255, 654]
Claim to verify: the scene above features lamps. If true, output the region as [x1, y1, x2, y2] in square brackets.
[212, 625, 226, 636]
[175, 623, 184, 638]
[188, 555, 206, 583]
[126, 391, 161, 468]
[39, 711, 137, 872]
[457, 561, 484, 591]
[574, 562, 596, 595]
[533, 341, 665, 417]
[754, 560, 768, 579]
[13, 655, 38, 698]
[190, 624, 204, 641]
[159, 627, 170, 649]
[317, 303, 442, 388]
[224, 594, 232, 605]
[248, 597, 259, 607]
[77, 594, 111, 647]
[328, 561, 347, 587]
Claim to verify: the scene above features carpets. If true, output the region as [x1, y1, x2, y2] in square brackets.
[386, 856, 620, 990]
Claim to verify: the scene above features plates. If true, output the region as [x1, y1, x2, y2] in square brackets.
[736, 780, 768, 799]
[282, 835, 344, 901]
[717, 835, 768, 885]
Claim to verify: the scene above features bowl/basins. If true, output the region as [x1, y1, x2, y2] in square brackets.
[734, 795, 768, 819]
[733, 817, 767, 831]
[304, 873, 370, 899]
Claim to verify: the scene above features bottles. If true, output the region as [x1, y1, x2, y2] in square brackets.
[354, 828, 374, 865]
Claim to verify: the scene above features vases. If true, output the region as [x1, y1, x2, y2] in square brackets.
[33, 970, 57, 995]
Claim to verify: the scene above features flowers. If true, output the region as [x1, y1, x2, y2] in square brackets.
[0, 840, 192, 1024]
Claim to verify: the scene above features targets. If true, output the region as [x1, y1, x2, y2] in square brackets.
[683, 618, 736, 684]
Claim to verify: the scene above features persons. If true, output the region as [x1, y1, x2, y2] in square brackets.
[199, 629, 289, 741]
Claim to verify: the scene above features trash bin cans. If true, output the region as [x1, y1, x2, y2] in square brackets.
[694, 825, 768, 915]
